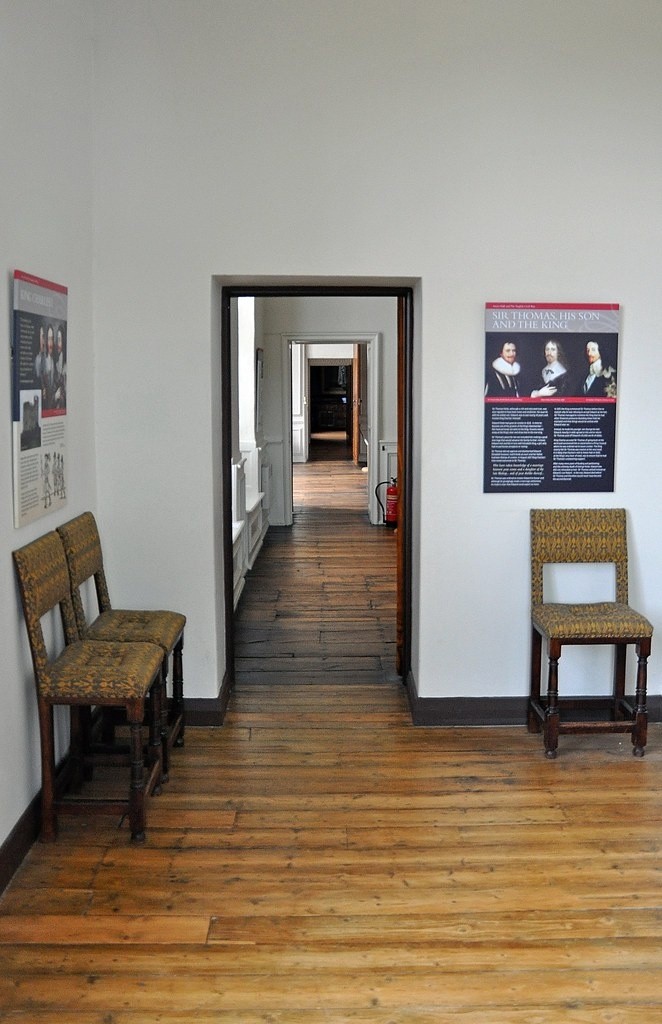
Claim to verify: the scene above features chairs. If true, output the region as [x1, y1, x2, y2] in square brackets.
[10, 529, 164, 846]
[57, 510, 187, 784]
[527, 508, 654, 759]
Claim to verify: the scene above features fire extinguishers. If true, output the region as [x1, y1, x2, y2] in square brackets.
[375, 476, 399, 526]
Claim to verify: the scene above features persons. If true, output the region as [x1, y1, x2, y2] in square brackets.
[34, 323, 45, 408]
[485, 338, 523, 397]
[45, 324, 55, 409]
[526, 336, 570, 398]
[574, 339, 617, 397]
[56, 325, 67, 408]
